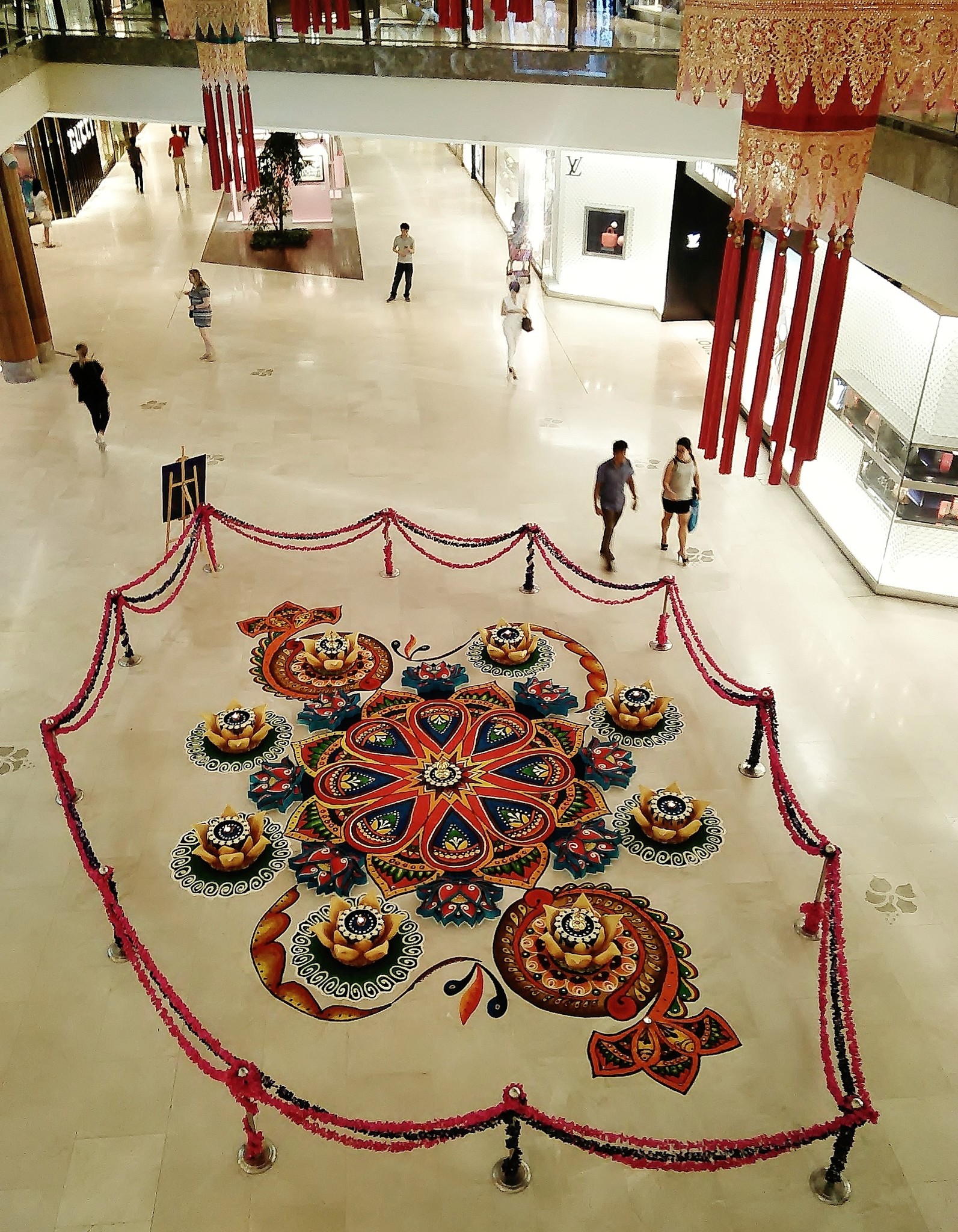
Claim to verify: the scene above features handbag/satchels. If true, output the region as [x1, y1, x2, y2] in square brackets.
[521, 317, 533, 332]
[687, 499, 699, 531]
[601, 227, 618, 248]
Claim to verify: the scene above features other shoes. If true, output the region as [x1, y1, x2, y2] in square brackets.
[405, 297, 411, 302]
[176, 186, 180, 191]
[185, 185, 189, 188]
[387, 297, 395, 302]
[200, 353, 216, 362]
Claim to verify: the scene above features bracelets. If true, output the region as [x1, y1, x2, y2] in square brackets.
[192, 304, 196, 310]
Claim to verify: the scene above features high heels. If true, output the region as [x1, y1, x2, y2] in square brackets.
[661, 543, 668, 551]
[678, 551, 689, 565]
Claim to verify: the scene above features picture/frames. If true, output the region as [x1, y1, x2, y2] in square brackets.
[580, 202, 634, 260]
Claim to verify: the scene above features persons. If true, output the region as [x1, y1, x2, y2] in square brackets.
[29, 178, 56, 248]
[593, 440, 637, 571]
[386, 223, 415, 302]
[501, 282, 533, 381]
[661, 437, 700, 563]
[126, 137, 146, 195]
[168, 127, 189, 190]
[68, 341, 111, 452]
[183, 268, 215, 363]
[508, 202, 529, 272]
[179, 125, 191, 145]
